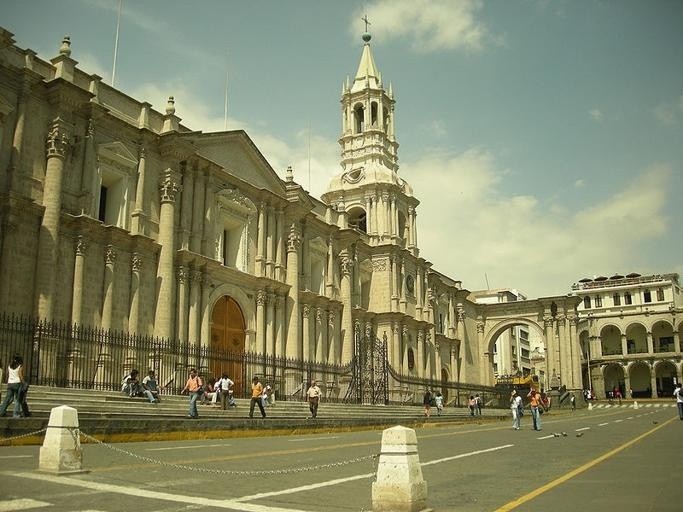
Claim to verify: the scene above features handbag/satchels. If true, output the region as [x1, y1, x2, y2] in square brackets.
[477, 397, 482, 407]
[538, 406, 544, 414]
[196, 376, 204, 396]
[519, 407, 524, 417]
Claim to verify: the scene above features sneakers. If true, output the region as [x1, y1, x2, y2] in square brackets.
[513, 426, 520, 430]
[470, 413, 482, 416]
[530, 427, 543, 431]
[129, 392, 137, 398]
[186, 414, 200, 418]
[437, 413, 443, 417]
[425, 412, 430, 418]
[152, 397, 160, 404]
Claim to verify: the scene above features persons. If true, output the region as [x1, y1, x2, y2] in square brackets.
[121, 369, 140, 397]
[0, 353, 32, 417]
[434, 393, 443, 416]
[672, 383, 683, 419]
[269, 384, 275, 407]
[526, 387, 546, 431]
[198, 372, 235, 409]
[180, 368, 203, 419]
[509, 388, 522, 429]
[140, 371, 159, 402]
[247, 376, 266, 419]
[474, 393, 481, 415]
[261, 381, 270, 406]
[305, 379, 322, 419]
[423, 388, 431, 417]
[570, 395, 576, 412]
[468, 395, 476, 415]
[546, 393, 552, 411]
[629, 386, 632, 398]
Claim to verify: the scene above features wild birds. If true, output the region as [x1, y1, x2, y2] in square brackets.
[553, 433, 560, 437]
[652, 421, 659, 425]
[576, 432, 584, 437]
[560, 431, 568, 437]
